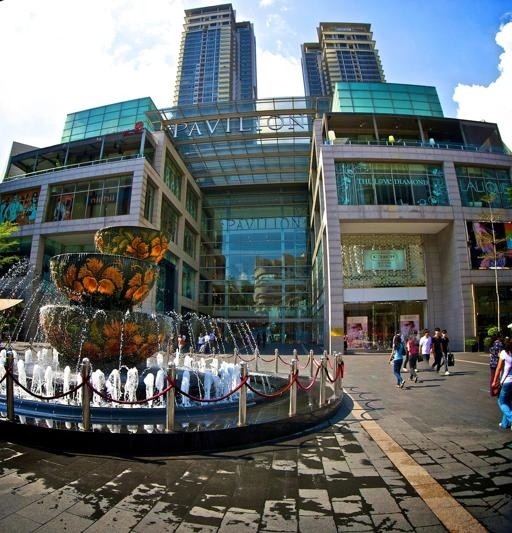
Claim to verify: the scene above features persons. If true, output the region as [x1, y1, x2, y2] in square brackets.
[388, 326, 454, 388]
[487, 330, 504, 396]
[402, 320, 417, 333]
[174, 326, 266, 356]
[349, 323, 363, 332]
[0, 189, 40, 225]
[472, 221, 506, 268]
[491, 327, 512, 433]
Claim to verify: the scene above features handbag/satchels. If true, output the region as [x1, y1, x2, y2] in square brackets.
[418, 355, 424, 362]
[402, 343, 406, 356]
[492, 382, 502, 398]
[448, 354, 455, 366]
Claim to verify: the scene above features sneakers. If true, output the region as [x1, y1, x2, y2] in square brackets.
[415, 368, 419, 372]
[409, 374, 418, 384]
[402, 367, 409, 372]
[445, 370, 451, 376]
[396, 379, 406, 389]
[432, 364, 440, 373]
[498, 423, 512, 432]
